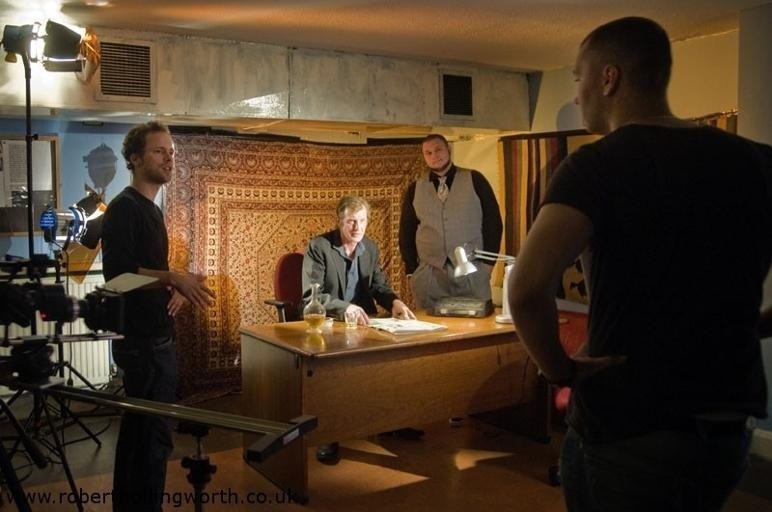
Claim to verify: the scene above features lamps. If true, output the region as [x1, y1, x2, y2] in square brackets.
[451, 240, 516, 324]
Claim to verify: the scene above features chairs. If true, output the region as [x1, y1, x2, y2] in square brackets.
[263, 252, 306, 321]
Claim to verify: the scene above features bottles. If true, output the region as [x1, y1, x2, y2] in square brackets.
[303, 282, 326, 331]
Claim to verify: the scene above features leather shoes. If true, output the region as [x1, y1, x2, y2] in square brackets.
[376, 428, 424, 440]
[449, 415, 463, 425]
[316, 442, 338, 461]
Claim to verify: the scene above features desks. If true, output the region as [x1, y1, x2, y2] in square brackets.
[238, 312, 572, 508]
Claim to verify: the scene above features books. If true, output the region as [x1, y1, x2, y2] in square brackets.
[363, 316, 445, 334]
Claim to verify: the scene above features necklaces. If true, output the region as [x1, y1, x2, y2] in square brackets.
[644, 114, 679, 120]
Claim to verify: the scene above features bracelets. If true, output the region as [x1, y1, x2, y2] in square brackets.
[542, 358, 574, 387]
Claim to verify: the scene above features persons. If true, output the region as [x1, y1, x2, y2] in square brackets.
[103, 121, 216, 512]
[300, 197, 424, 460]
[399, 134, 503, 427]
[508, 14, 772, 512]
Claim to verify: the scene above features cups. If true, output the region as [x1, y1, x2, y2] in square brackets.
[344, 310, 358, 330]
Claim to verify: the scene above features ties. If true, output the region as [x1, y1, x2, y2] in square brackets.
[437, 176, 449, 203]
